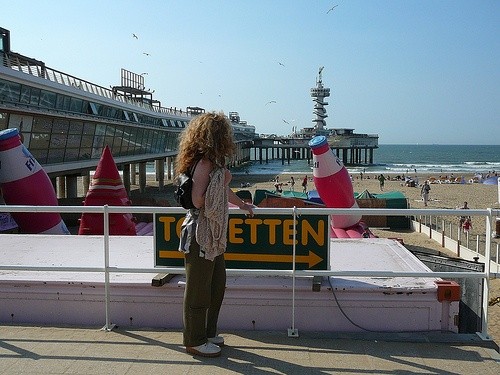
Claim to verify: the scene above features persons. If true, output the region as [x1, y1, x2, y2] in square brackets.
[238, 168, 500, 241]
[176, 114, 257, 356]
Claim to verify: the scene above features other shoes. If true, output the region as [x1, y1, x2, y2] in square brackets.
[207, 336, 224, 346]
[186, 342, 222, 357]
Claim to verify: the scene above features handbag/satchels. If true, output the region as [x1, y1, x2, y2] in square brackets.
[174, 157, 222, 209]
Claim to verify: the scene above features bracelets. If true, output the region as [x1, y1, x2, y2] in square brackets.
[239, 201, 244, 208]
